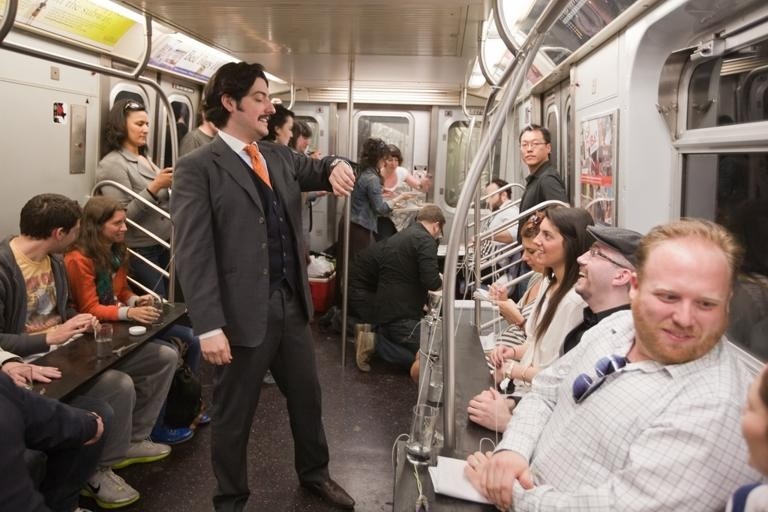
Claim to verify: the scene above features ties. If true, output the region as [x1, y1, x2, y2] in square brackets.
[243, 144, 275, 191]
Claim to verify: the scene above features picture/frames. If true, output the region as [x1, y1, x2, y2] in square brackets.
[578, 106, 620, 227]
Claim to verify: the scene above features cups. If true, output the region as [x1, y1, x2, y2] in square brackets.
[425, 293, 442, 322]
[93, 322, 115, 359]
[407, 404, 438, 464]
[9, 365, 34, 392]
[148, 294, 163, 327]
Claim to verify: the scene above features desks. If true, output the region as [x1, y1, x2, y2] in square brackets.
[1, 297, 191, 409]
[392, 315, 510, 512]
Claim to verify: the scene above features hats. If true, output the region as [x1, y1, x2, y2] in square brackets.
[585, 221, 645, 270]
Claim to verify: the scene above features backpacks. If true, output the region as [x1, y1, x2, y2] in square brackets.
[164, 333, 203, 427]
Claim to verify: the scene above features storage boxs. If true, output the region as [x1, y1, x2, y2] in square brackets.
[307, 269, 341, 314]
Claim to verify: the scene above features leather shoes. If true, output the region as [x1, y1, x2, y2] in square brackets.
[300, 476, 354, 508]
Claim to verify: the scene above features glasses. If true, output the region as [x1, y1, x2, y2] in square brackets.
[123, 101, 146, 111]
[519, 141, 548, 149]
[570, 349, 632, 406]
[588, 248, 631, 269]
[436, 223, 446, 241]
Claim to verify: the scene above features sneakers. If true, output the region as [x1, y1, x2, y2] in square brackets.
[79, 467, 140, 509]
[151, 424, 194, 444]
[96, 439, 173, 469]
[354, 323, 375, 373]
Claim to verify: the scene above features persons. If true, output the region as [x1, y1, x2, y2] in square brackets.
[266, 102, 333, 266]
[170, 62, 355, 511]
[1, 100, 215, 510]
[463, 123, 767, 512]
[337, 137, 445, 383]
